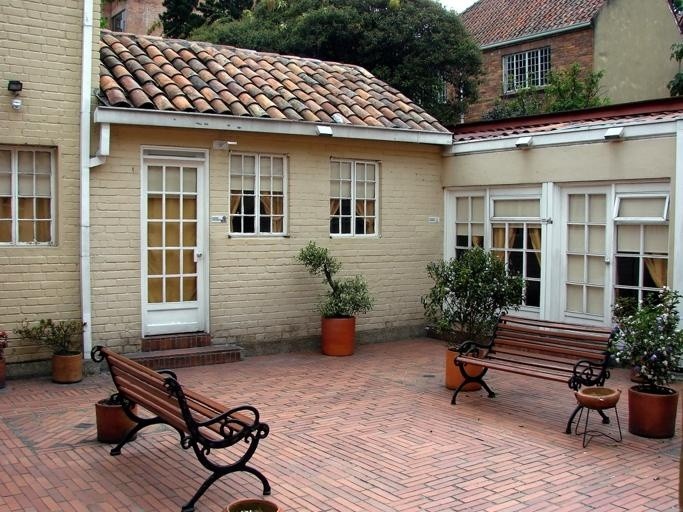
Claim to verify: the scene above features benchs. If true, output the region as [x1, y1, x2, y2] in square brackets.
[85, 345, 295, 511]
[450, 313, 617, 434]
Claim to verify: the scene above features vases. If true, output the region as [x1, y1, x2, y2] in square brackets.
[92, 390, 137, 444]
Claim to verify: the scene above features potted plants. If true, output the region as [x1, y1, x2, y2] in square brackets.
[606, 289, 679, 438]
[13, 311, 87, 384]
[420, 248, 529, 391]
[294, 240, 373, 358]
[0, 331, 9, 388]
